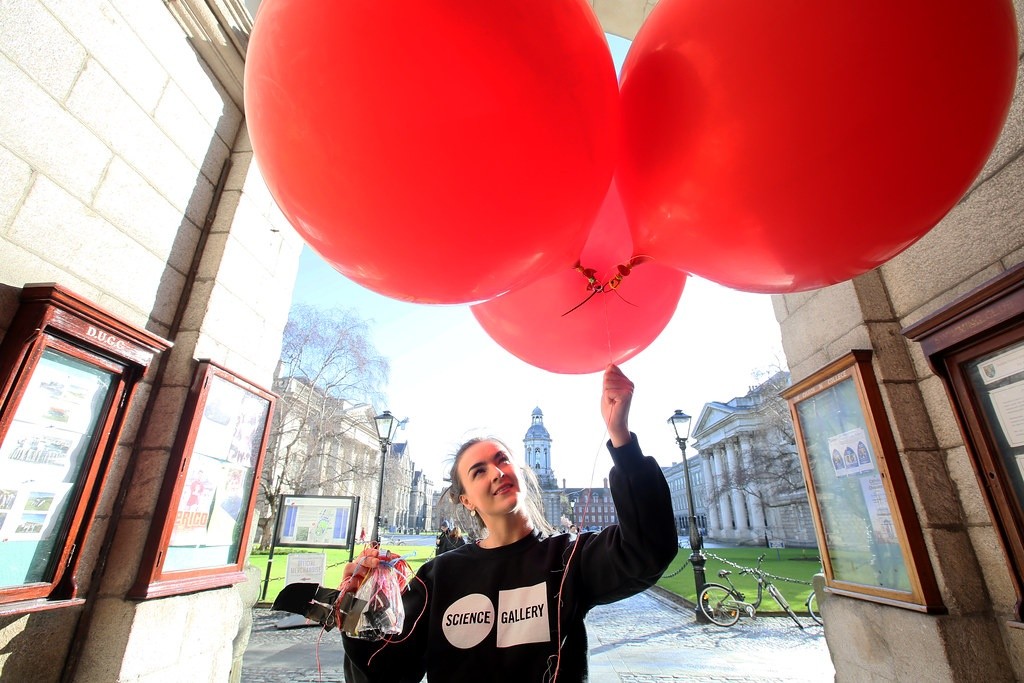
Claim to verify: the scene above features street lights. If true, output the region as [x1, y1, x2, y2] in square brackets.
[371, 410, 400, 550]
[667, 409, 715, 625]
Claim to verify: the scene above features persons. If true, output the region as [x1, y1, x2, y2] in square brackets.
[551, 525, 562, 538]
[398, 525, 402, 533]
[557, 526, 566, 534]
[436, 519, 452, 556]
[566, 524, 578, 542]
[441, 526, 466, 554]
[337, 363, 679, 683]
[359, 526, 366, 544]
[415, 526, 419, 535]
[263, 534, 272, 551]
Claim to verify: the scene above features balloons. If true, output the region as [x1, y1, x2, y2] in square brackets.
[469, 175, 687, 375]
[242, 0, 619, 308]
[617, 1, 1020, 295]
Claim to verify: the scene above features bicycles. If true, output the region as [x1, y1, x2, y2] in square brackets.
[805, 555, 824, 625]
[699, 552, 803, 630]
